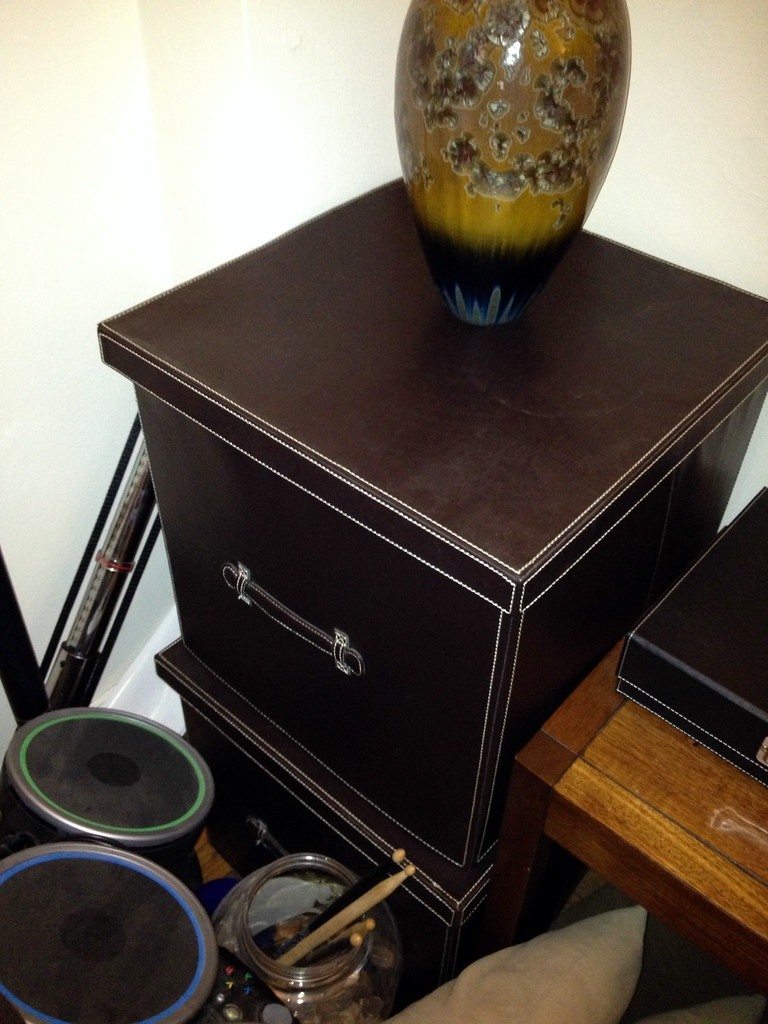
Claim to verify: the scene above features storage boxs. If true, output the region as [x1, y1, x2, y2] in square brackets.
[97, 178, 768, 1019]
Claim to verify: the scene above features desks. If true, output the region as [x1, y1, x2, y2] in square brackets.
[480, 634, 767, 999]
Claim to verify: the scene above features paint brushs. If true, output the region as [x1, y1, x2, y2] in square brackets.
[268, 850, 416, 967]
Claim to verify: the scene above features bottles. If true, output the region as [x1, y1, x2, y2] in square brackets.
[212, 851, 402, 1024]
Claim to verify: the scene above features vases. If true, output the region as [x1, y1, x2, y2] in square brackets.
[393, 0, 633, 336]
